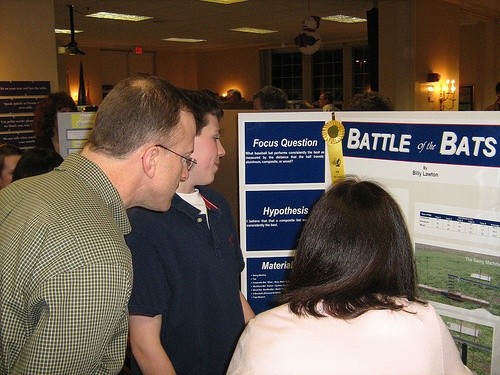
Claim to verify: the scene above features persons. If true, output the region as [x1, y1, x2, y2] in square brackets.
[0, 73, 199, 375]
[225, 173, 476, 375]
[0, 87, 395, 188]
[124, 88, 256, 375]
[486, 83, 500, 110]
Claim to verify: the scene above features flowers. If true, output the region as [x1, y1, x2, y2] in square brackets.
[322, 120, 345, 144]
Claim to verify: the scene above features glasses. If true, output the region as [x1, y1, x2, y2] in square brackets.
[154, 144, 197, 170]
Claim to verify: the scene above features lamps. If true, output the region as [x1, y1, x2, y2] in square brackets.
[427, 79, 456, 111]
